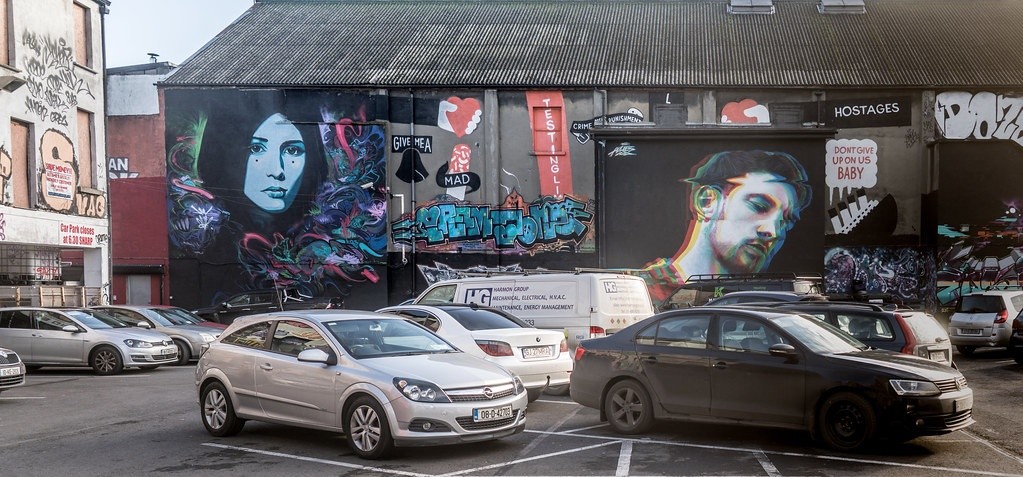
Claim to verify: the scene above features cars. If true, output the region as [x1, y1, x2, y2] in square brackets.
[1006, 308, 1023, 369]
[373, 303, 572, 406]
[569, 305, 976, 456]
[949, 285, 1023, 356]
[701, 289, 827, 306]
[1, 298, 232, 390]
[196, 308, 528, 461]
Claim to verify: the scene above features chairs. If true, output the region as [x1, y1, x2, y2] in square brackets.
[344, 318, 899, 362]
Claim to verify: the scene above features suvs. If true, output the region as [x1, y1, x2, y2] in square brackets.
[655, 273, 825, 313]
[737, 300, 952, 372]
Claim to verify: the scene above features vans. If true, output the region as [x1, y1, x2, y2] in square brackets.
[412, 271, 657, 396]
